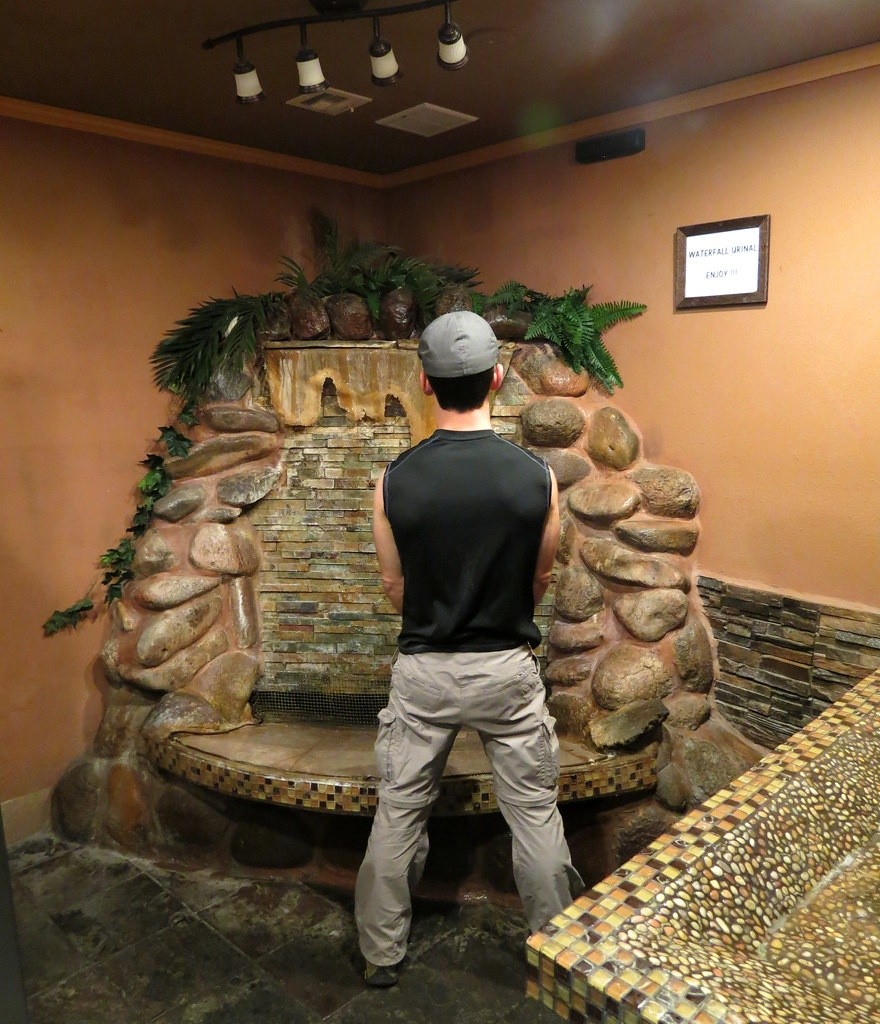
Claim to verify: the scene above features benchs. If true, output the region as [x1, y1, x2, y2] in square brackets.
[145, 714, 652, 813]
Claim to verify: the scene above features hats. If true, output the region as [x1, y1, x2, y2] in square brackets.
[417, 311, 498, 378]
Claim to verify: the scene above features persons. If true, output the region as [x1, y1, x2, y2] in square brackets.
[355, 311, 588, 988]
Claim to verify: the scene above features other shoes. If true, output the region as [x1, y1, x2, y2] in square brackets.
[363, 959, 400, 986]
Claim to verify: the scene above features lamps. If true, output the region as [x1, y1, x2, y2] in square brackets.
[202, 0, 471, 106]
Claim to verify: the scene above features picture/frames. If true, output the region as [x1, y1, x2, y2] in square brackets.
[675, 213, 769, 308]
